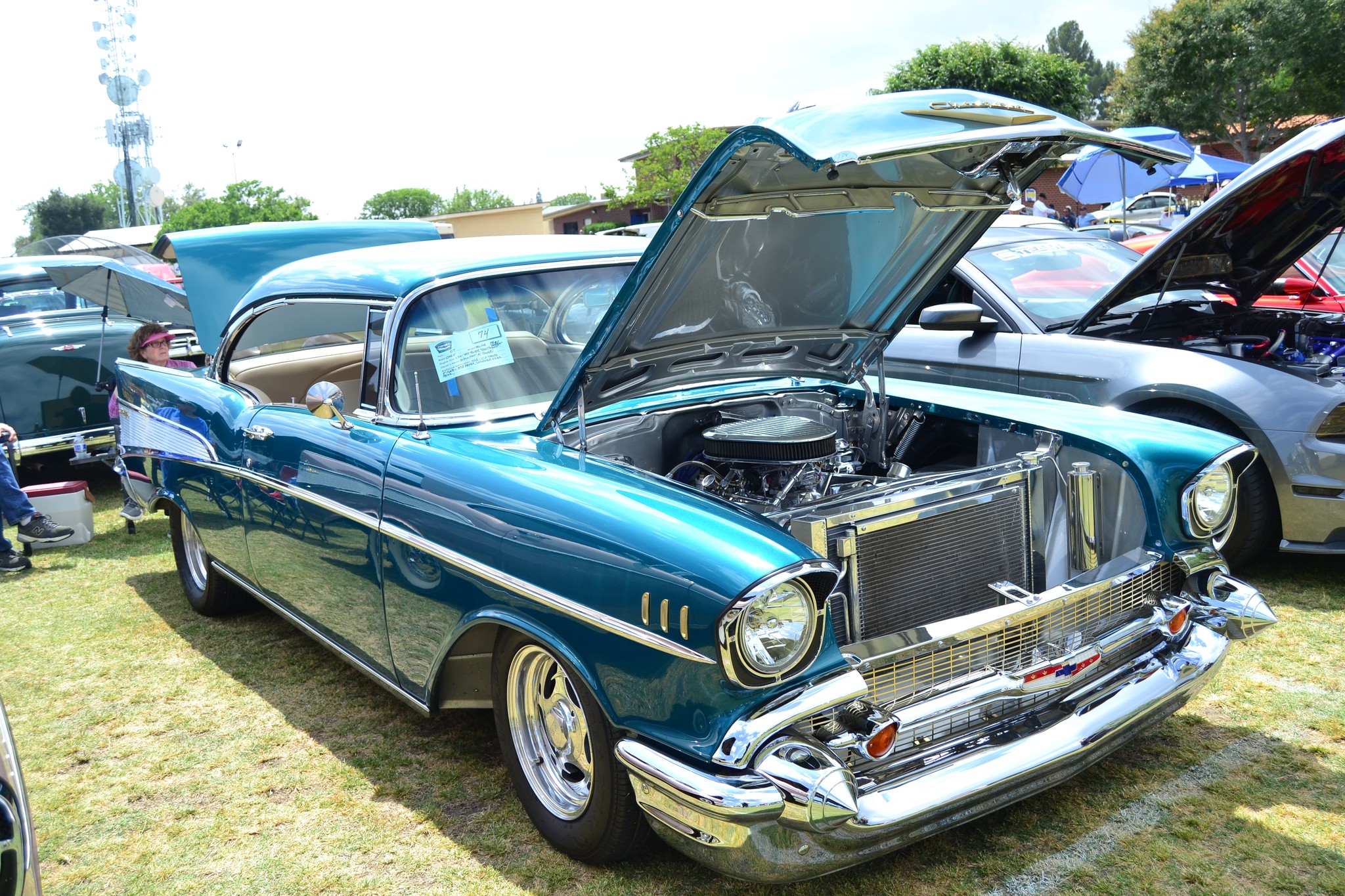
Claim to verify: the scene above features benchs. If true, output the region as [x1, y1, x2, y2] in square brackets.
[401, 350, 582, 414]
[229, 331, 549, 413]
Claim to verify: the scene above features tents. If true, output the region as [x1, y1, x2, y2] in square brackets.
[1162, 153, 1254, 219]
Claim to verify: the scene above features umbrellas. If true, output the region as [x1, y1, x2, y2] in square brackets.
[42, 260, 196, 391]
[1055, 127, 1195, 241]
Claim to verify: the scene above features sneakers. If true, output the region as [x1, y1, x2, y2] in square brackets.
[119, 496, 147, 521]
[16, 512, 75, 544]
[0, 551, 31, 573]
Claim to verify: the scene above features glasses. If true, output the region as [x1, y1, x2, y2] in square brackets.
[141, 339, 170, 348]
[1063, 211, 1068, 213]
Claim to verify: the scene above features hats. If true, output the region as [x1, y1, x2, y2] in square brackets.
[140, 332, 175, 347]
[1162, 206, 1173, 214]
[1039, 192, 1047, 199]
[1079, 206, 1087, 211]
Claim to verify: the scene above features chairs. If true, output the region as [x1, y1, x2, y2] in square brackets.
[0, 432, 32, 555]
[152, 455, 330, 542]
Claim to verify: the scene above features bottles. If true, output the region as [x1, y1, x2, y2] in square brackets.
[73, 434, 87, 459]
[1176, 195, 1202, 214]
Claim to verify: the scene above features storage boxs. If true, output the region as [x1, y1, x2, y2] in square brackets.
[19, 481, 94, 549]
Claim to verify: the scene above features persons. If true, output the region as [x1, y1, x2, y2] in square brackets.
[1007, 199, 1028, 215]
[1160, 206, 1175, 219]
[108, 323, 198, 538]
[1204, 179, 1232, 202]
[0, 423, 75, 571]
[1033, 193, 1061, 218]
[1075, 206, 1099, 228]
[1059, 205, 1077, 228]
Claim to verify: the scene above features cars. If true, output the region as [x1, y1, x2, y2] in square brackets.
[0, 201, 1344, 490]
[897, 117, 1345, 578]
[107, 85, 1281, 889]
